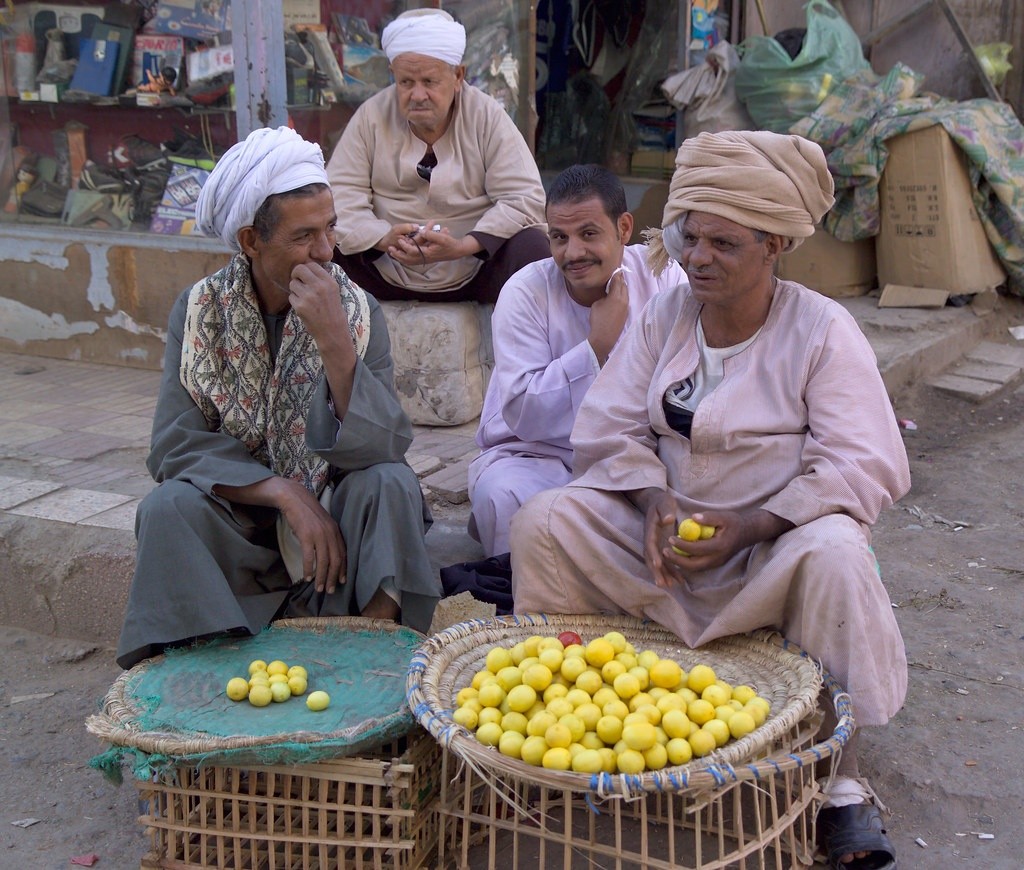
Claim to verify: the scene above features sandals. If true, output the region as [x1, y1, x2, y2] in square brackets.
[811, 804, 897, 870]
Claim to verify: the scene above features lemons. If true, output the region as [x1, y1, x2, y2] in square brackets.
[670, 518, 714, 556]
[452, 632, 770, 775]
[226, 660, 330, 710]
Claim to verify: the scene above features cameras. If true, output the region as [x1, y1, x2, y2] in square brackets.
[409, 224, 440, 237]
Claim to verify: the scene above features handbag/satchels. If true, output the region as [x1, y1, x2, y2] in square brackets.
[20, 177, 69, 218]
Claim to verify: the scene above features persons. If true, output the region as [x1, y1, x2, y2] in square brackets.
[464, 128, 914, 870]
[115, 125, 442, 670]
[324, 7, 552, 304]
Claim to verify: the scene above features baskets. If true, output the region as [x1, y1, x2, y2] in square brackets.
[139, 726, 456, 870]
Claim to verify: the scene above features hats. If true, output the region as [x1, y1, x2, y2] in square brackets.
[195, 125, 332, 249]
[381, 9, 467, 66]
[661, 130, 837, 263]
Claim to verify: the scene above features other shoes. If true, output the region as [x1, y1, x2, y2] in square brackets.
[138, 769, 212, 832]
[81, 132, 230, 228]
[451, 774, 519, 835]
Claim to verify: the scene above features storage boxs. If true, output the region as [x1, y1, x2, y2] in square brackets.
[780, 215, 868, 298]
[875, 124, 1006, 298]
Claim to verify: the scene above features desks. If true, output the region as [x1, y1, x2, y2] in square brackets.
[11, 101, 355, 170]
